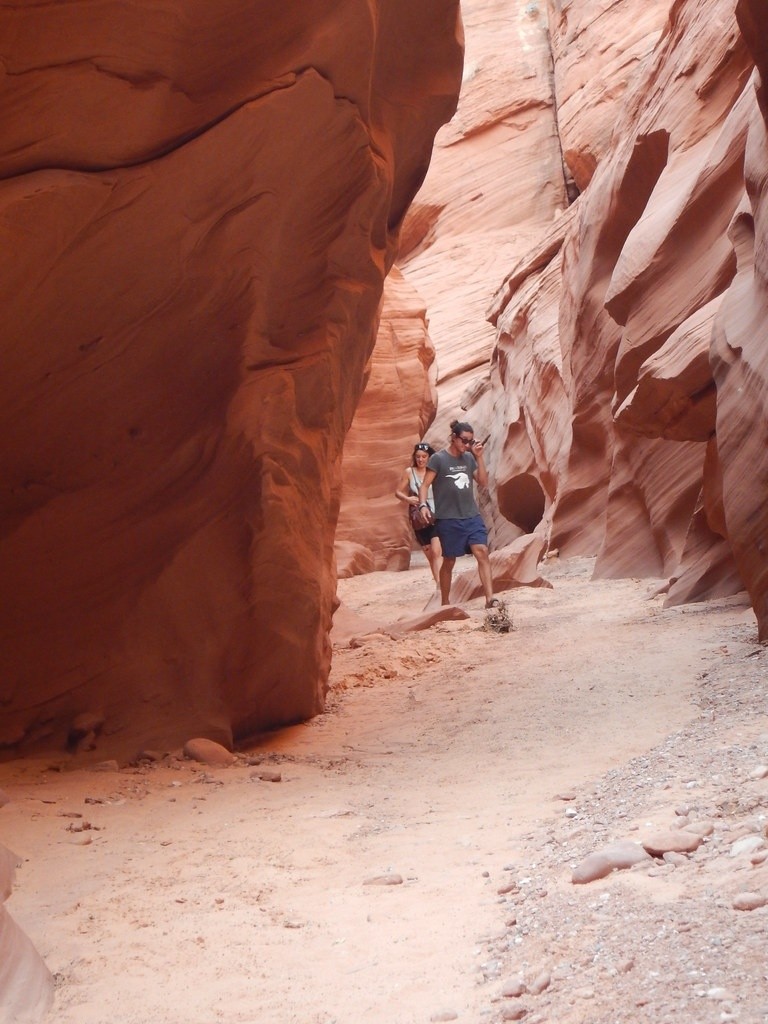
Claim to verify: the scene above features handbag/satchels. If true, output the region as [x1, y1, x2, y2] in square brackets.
[408, 467, 436, 529]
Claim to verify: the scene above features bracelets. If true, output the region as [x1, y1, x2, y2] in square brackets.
[419, 503, 427, 511]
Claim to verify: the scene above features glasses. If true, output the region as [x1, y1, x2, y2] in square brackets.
[415, 444, 431, 453]
[455, 434, 475, 446]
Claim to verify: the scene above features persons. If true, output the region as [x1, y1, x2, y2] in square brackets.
[422, 421, 499, 609]
[394, 442, 442, 586]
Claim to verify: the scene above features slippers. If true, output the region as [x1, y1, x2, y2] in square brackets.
[484, 599, 500, 609]
